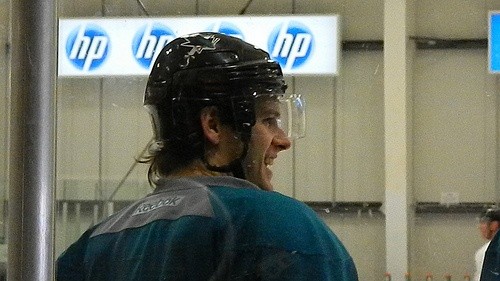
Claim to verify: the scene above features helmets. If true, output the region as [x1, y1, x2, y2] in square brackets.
[480, 204, 499, 239]
[144, 32, 306, 140]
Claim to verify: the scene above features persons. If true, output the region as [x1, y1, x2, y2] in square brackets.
[474, 207, 500, 281]
[55, 32, 359, 281]
